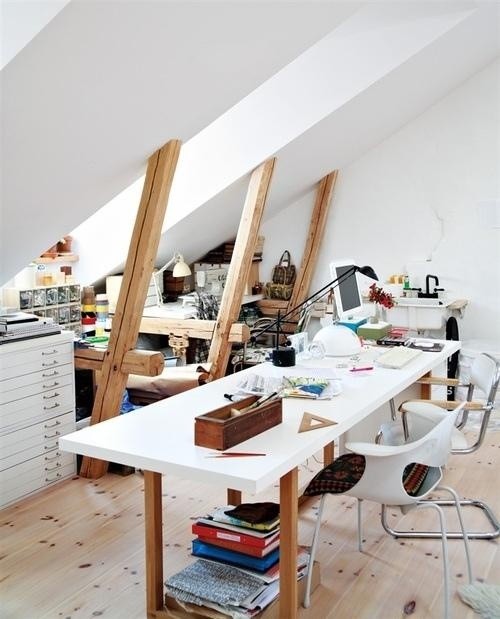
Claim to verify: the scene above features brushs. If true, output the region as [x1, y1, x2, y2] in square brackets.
[231, 391, 277, 418]
[259, 376, 292, 407]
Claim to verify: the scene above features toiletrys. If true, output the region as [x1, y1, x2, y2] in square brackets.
[403, 272, 409, 288]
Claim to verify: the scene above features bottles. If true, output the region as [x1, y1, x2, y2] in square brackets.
[252, 281, 262, 295]
[327, 287, 335, 305]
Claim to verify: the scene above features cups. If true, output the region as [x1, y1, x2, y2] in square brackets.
[326, 304, 334, 314]
[44, 265, 76, 285]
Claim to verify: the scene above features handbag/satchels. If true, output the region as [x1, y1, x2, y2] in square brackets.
[273, 250, 295, 284]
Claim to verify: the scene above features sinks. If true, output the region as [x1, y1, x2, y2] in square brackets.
[383, 298, 456, 332]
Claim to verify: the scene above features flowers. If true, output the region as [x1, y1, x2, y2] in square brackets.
[368, 283, 398, 309]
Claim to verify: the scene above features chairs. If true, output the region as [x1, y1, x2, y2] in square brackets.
[231, 300, 315, 375]
[302, 398, 478, 619]
[376, 354, 498, 543]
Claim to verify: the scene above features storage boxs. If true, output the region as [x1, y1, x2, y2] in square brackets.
[165, 561, 321, 619]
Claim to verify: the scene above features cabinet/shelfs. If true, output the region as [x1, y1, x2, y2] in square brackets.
[0, 330, 78, 512]
[2, 282, 82, 339]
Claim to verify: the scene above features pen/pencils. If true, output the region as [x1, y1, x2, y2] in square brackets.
[350, 367, 374, 371]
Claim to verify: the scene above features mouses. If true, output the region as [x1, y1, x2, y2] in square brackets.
[329, 260, 363, 322]
[414, 341, 434, 347]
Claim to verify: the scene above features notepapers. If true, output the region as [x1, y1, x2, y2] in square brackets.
[388, 328, 407, 337]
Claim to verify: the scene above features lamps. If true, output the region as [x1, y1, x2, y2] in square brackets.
[250, 265, 378, 367]
[152, 252, 192, 311]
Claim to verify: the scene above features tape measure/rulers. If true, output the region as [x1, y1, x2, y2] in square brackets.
[298, 412, 338, 433]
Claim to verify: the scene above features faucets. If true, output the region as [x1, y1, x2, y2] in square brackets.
[425, 274, 440, 293]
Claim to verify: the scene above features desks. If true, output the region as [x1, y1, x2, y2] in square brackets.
[58, 336, 462, 619]
[108, 294, 265, 359]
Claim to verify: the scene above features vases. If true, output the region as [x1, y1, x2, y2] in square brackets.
[46, 235, 72, 254]
[373, 301, 387, 322]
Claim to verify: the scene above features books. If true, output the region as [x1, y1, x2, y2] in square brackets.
[190, 505, 312, 585]
[0, 315, 38, 325]
[377, 335, 410, 346]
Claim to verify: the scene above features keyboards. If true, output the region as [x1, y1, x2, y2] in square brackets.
[373, 345, 423, 369]
